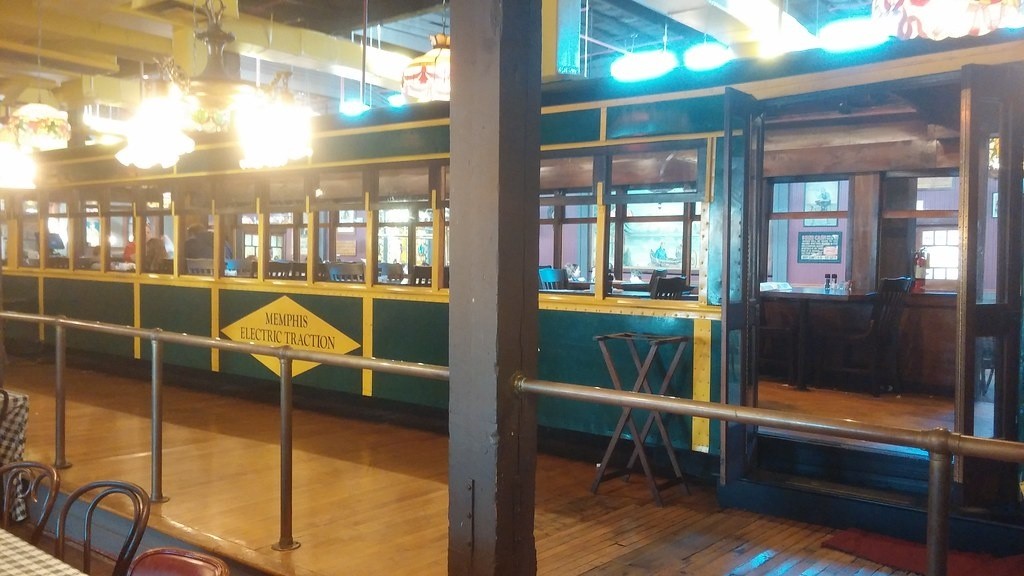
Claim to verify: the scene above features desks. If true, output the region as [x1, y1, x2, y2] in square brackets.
[760, 281, 852, 391]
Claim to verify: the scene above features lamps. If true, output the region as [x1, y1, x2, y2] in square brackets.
[9, 29, 70, 151]
[0, 123, 39, 190]
[110, 86, 197, 170]
[400, 0, 451, 102]
[229, 73, 318, 172]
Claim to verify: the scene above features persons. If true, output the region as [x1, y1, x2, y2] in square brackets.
[123, 222, 233, 275]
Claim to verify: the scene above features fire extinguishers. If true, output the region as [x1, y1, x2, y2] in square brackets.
[909, 246, 927, 293]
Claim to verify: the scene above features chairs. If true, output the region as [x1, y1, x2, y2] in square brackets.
[56, 480, 150, 576]
[647, 269, 668, 292]
[291, 262, 326, 280]
[414, 265, 432, 286]
[0, 460, 60, 546]
[326, 262, 365, 283]
[128, 547, 231, 576]
[650, 275, 689, 300]
[0, 388, 30, 521]
[381, 263, 404, 281]
[186, 258, 214, 275]
[226, 258, 250, 272]
[538, 268, 570, 290]
[814, 277, 916, 392]
[250, 261, 291, 279]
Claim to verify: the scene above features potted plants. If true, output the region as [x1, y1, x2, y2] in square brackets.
[608, 274, 613, 294]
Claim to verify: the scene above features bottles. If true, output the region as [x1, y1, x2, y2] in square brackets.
[825, 274, 830, 287]
[832, 274, 837, 287]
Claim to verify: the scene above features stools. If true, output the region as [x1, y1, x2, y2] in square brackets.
[590, 331, 692, 508]
